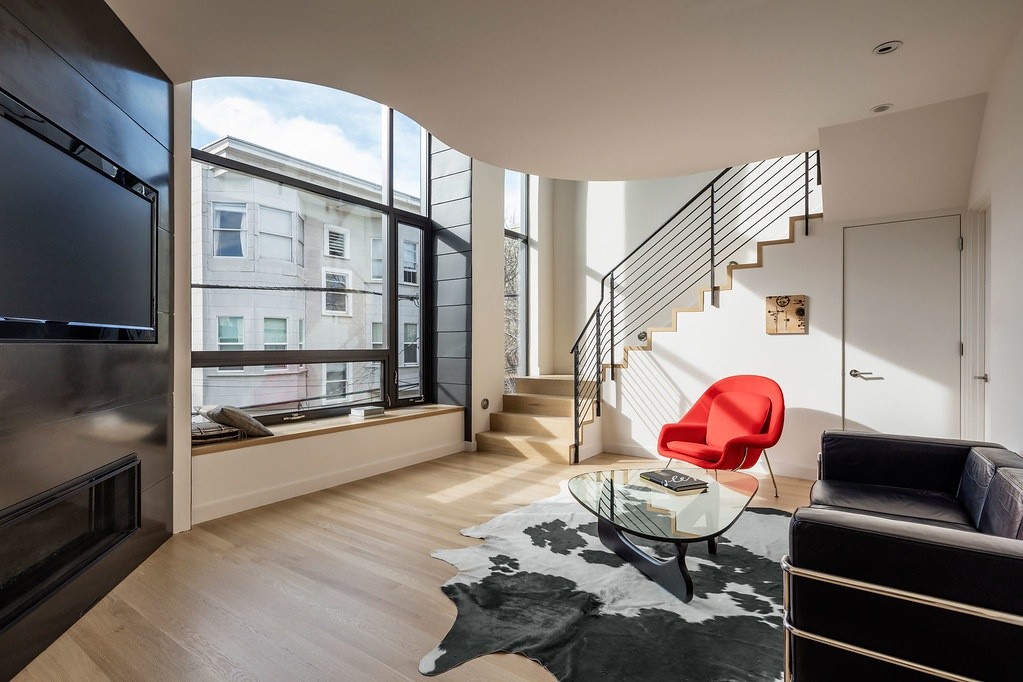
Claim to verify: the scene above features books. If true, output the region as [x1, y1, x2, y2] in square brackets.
[640, 468, 709, 496]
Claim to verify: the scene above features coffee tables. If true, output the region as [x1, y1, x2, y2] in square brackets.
[567, 468, 759, 602]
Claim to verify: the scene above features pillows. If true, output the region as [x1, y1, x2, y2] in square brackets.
[193, 405, 274, 437]
[192, 415, 246, 444]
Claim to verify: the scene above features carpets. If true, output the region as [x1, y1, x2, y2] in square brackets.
[419, 477, 793, 682]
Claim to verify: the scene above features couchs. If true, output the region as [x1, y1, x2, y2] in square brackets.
[780, 428, 1023, 682]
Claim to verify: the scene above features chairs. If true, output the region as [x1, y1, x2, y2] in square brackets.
[658, 376, 785, 498]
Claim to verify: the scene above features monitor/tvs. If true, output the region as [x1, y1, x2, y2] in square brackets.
[0, 91, 158, 344]
